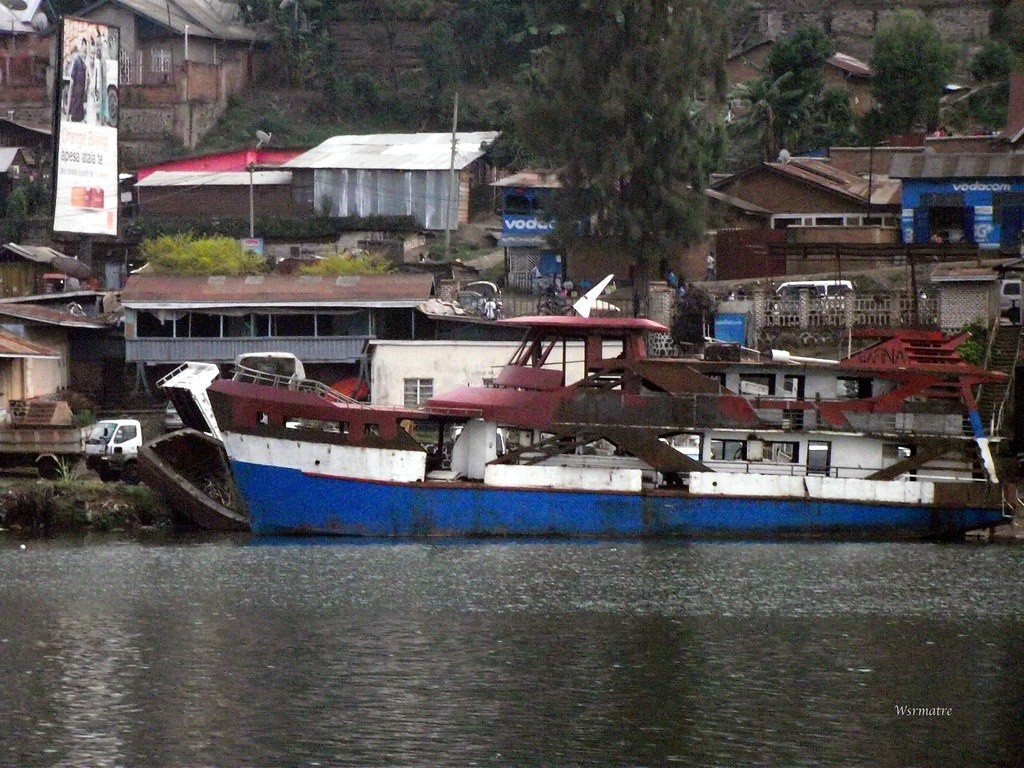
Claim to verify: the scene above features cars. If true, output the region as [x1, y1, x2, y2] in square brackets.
[162, 401, 185, 432]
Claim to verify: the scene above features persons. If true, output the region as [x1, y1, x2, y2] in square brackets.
[547, 273, 574, 298]
[677, 278, 687, 298]
[929, 231, 944, 260]
[66, 38, 100, 123]
[419, 253, 425, 264]
[485, 298, 497, 321]
[704, 250, 716, 281]
[920, 291, 927, 299]
[728, 286, 746, 301]
[666, 268, 677, 288]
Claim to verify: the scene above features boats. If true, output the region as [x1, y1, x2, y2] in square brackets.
[206, 319, 1015, 541]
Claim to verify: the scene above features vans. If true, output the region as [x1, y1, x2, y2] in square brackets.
[773, 280, 853, 313]
[1000, 279, 1022, 322]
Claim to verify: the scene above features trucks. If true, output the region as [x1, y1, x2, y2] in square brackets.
[85, 360, 223, 484]
[0, 407, 98, 481]
